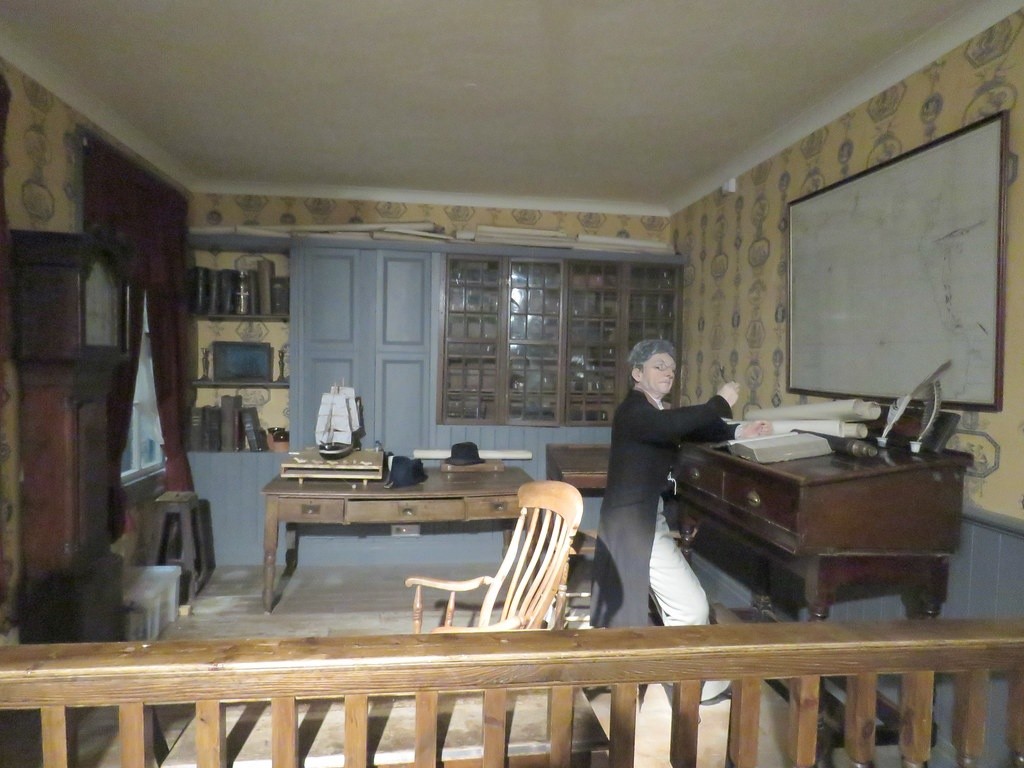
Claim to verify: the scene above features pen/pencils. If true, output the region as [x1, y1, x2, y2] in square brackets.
[716, 363, 731, 382]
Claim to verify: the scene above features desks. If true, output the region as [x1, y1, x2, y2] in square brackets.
[261, 467, 546, 615]
[158, 683, 609, 768]
[545, 444, 611, 610]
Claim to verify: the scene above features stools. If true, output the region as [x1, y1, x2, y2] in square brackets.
[149, 491, 209, 603]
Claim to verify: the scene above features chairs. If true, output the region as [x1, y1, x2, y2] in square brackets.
[405, 479, 584, 633]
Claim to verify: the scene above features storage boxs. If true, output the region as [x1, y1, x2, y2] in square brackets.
[121, 565, 181, 642]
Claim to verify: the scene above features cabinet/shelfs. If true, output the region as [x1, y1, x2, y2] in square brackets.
[12, 227, 133, 573]
[672, 443, 976, 768]
[180, 232, 293, 456]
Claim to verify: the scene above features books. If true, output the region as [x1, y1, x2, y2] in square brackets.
[704, 431, 836, 464]
[189, 259, 291, 453]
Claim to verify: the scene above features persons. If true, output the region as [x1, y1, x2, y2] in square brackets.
[588, 339, 775, 701]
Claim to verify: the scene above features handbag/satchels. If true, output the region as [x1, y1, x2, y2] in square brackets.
[384, 456, 428, 489]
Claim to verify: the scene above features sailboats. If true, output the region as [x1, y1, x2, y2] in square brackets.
[315, 380, 361, 459]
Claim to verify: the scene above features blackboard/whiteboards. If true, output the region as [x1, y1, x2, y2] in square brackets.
[783, 112, 1011, 410]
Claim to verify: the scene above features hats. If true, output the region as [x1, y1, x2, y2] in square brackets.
[445, 443, 485, 466]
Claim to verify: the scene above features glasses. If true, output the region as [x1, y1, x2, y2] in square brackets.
[636, 362, 679, 376]
